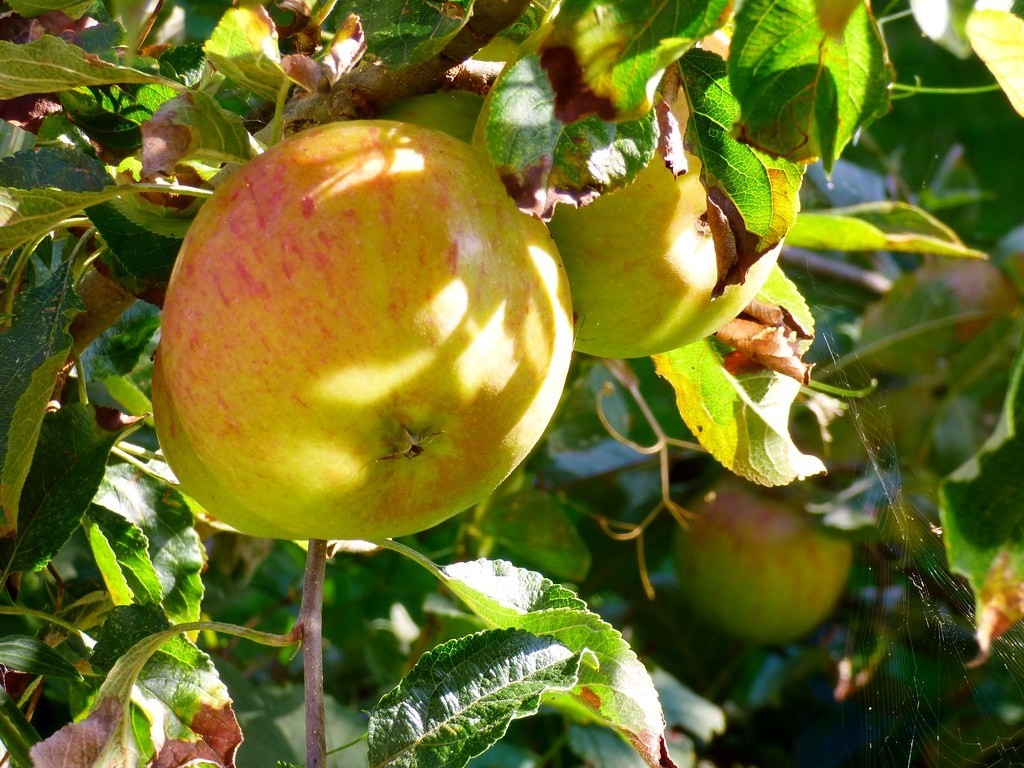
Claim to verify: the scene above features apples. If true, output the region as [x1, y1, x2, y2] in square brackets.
[148, 29, 783, 545]
[679, 475, 854, 639]
